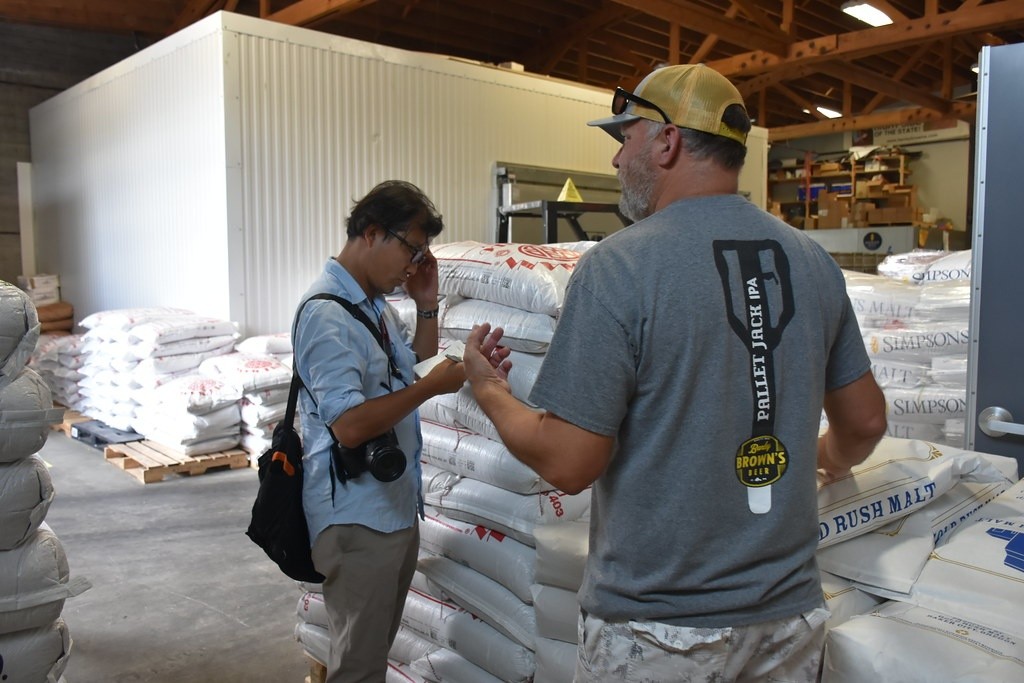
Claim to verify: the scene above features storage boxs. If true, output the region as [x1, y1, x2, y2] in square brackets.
[16, 273, 60, 307]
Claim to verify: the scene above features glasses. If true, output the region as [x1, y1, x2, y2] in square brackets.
[380, 221, 425, 266]
[612, 86, 671, 127]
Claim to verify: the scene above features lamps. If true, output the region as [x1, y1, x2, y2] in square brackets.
[816, 103, 844, 119]
[840, 0, 894, 28]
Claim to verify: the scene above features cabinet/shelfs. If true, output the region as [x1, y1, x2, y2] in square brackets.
[767, 151, 922, 228]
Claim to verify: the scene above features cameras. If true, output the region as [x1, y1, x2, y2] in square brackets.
[331, 425, 407, 482]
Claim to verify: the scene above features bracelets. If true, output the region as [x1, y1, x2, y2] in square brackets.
[415, 308, 439, 319]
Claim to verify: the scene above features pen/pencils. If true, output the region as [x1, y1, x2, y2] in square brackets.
[445, 353, 463, 364]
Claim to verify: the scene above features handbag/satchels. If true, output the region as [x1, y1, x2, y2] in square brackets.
[246, 426, 327, 585]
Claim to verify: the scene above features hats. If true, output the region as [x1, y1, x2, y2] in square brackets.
[587, 63, 751, 146]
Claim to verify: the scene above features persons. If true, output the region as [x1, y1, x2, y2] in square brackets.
[465, 64, 886, 683]
[291, 179, 467, 683]
